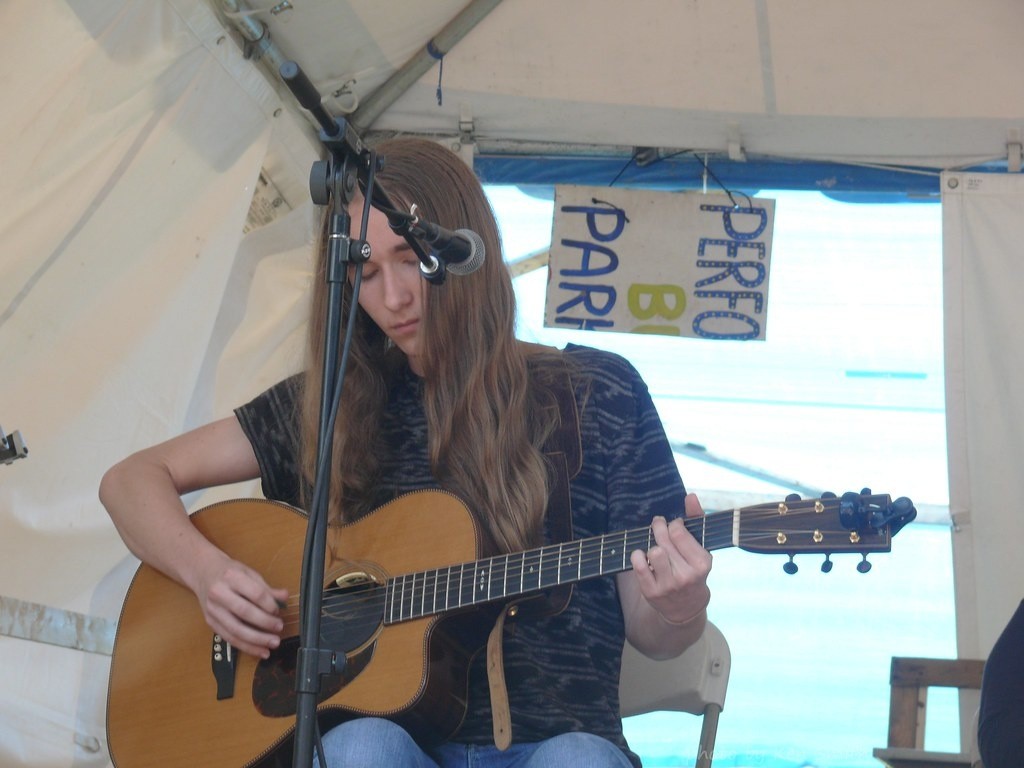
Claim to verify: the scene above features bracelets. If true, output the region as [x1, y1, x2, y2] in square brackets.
[656, 584, 711, 627]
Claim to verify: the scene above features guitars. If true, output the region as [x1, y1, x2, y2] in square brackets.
[104, 486, 916, 767]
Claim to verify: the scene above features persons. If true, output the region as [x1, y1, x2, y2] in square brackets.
[96, 139, 715, 768]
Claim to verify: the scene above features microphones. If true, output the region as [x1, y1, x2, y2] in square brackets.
[411, 216, 486, 275]
